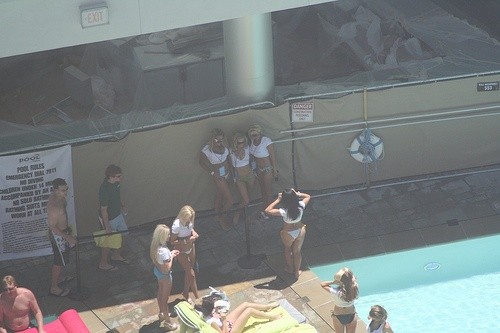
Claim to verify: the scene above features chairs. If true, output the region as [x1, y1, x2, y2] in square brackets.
[174, 301, 318, 333]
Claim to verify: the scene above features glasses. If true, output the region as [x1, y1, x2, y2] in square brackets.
[3, 286, 14, 292]
[215, 139, 225, 143]
[62, 187, 68, 192]
[238, 141, 245, 144]
[113, 175, 121, 178]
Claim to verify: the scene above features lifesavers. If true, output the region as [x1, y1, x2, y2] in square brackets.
[350, 135, 383, 163]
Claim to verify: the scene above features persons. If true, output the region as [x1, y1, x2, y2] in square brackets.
[201, 296, 284, 333]
[46, 178, 78, 297]
[264, 188, 310, 279]
[199, 125, 279, 231]
[366, 305, 395, 333]
[98, 164, 129, 272]
[150, 224, 180, 331]
[0, 275, 45, 333]
[170, 204, 200, 305]
[321, 269, 359, 333]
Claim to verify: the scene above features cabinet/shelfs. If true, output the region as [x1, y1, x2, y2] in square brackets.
[133, 43, 226, 110]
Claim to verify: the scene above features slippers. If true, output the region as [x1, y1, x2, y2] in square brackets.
[49, 288, 71, 297]
[98, 265, 121, 273]
[57, 276, 74, 286]
[112, 259, 131, 265]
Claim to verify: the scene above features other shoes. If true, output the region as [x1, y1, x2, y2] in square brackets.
[181, 295, 194, 305]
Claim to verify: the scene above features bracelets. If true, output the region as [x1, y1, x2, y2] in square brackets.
[184, 239, 188, 246]
[210, 171, 215, 177]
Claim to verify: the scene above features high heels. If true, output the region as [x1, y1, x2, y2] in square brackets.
[158, 313, 171, 321]
[164, 322, 178, 331]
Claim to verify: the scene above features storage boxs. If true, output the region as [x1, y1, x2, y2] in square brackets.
[64, 65, 93, 106]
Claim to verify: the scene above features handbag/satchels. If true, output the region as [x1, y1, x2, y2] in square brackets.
[93, 230, 121, 249]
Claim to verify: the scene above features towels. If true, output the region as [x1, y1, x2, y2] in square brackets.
[93, 230, 122, 249]
[175, 301, 299, 333]
[280, 323, 318, 333]
